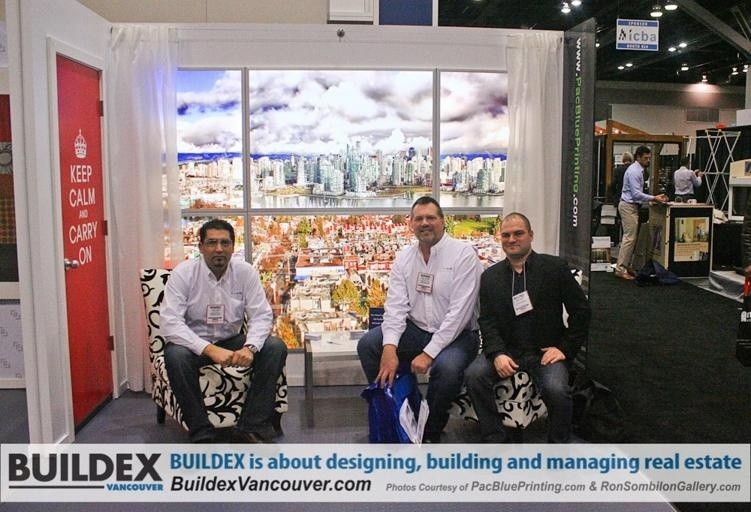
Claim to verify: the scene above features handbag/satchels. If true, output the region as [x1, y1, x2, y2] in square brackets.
[361, 372, 431, 445]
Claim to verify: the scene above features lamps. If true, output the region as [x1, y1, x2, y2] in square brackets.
[649, 0, 680, 18]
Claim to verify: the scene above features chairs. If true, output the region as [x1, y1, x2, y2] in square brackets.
[447, 266, 588, 444]
[139, 268, 287, 443]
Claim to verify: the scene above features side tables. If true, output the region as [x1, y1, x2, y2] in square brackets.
[302, 330, 377, 428]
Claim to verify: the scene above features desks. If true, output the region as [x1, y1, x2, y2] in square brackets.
[648, 203, 714, 281]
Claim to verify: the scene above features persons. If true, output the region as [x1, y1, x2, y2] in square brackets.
[616, 153, 632, 204]
[696, 226, 701, 241]
[682, 232, 686, 242]
[159, 220, 287, 443]
[614, 146, 664, 280]
[675, 159, 703, 199]
[463, 213, 592, 443]
[357, 197, 484, 443]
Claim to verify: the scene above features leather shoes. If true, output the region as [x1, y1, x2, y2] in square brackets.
[236, 430, 272, 444]
[615, 264, 637, 280]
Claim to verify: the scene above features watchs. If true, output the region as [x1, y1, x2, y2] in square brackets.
[246, 344, 257, 353]
